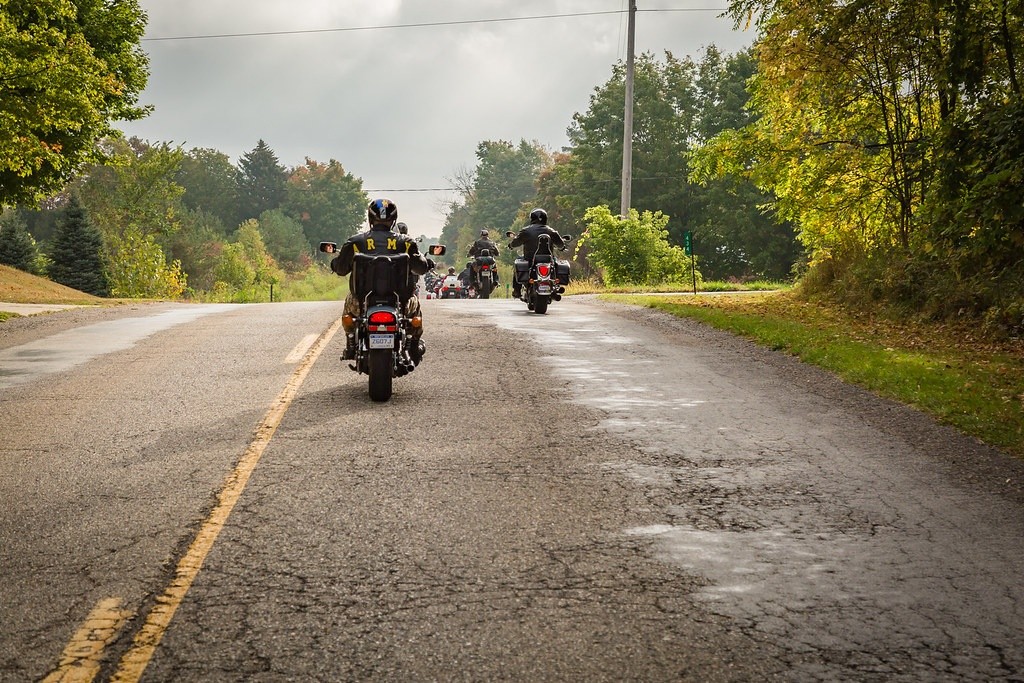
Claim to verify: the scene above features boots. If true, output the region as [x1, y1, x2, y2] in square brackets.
[342, 333, 357, 360]
[406, 332, 426, 358]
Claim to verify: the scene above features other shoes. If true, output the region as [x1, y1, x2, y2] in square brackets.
[512, 289, 522, 298]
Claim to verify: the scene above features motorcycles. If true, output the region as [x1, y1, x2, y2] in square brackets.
[424, 272, 463, 299]
[506, 231, 573, 315]
[457, 278, 480, 299]
[466, 243, 501, 299]
[319, 241, 447, 403]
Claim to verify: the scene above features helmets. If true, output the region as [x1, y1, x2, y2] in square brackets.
[480, 230, 489, 238]
[368, 200, 397, 232]
[448, 266, 455, 273]
[530, 208, 548, 225]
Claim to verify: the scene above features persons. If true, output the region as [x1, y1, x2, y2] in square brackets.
[424, 268, 437, 281]
[469, 230, 499, 287]
[434, 246, 442, 254]
[330, 199, 436, 355]
[443, 266, 456, 282]
[508, 209, 566, 298]
[457, 262, 477, 296]
[325, 245, 333, 253]
